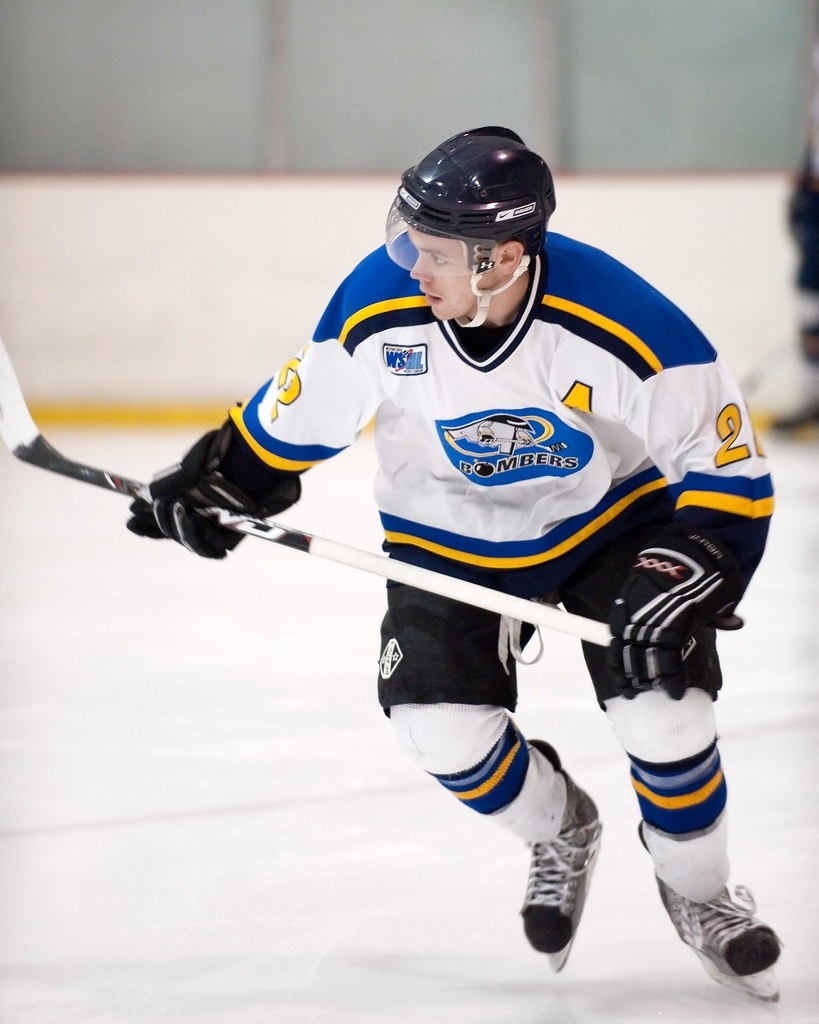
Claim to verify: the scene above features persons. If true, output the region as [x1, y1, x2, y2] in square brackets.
[128, 124, 786, 1005]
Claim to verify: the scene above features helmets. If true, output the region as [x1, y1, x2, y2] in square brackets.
[396, 125, 556, 276]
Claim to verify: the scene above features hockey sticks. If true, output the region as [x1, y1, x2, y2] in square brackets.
[0, 334, 614, 648]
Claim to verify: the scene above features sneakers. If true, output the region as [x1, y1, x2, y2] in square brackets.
[637, 820, 783, 1001]
[520, 739, 602, 973]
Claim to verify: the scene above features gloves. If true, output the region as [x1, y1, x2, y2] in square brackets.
[126, 418, 304, 560]
[609, 531, 743, 702]
